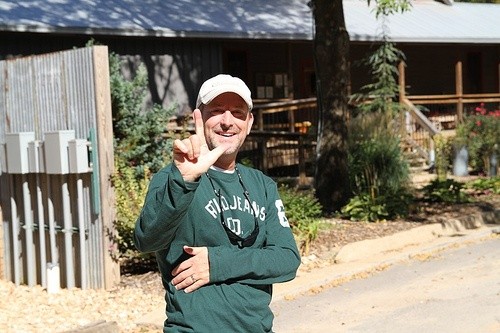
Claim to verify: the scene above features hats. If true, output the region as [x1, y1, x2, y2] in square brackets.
[196, 74, 253, 111]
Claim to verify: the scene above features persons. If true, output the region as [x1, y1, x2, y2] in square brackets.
[132, 74, 301, 333]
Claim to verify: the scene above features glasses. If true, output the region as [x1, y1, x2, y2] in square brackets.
[218, 188, 259, 248]
